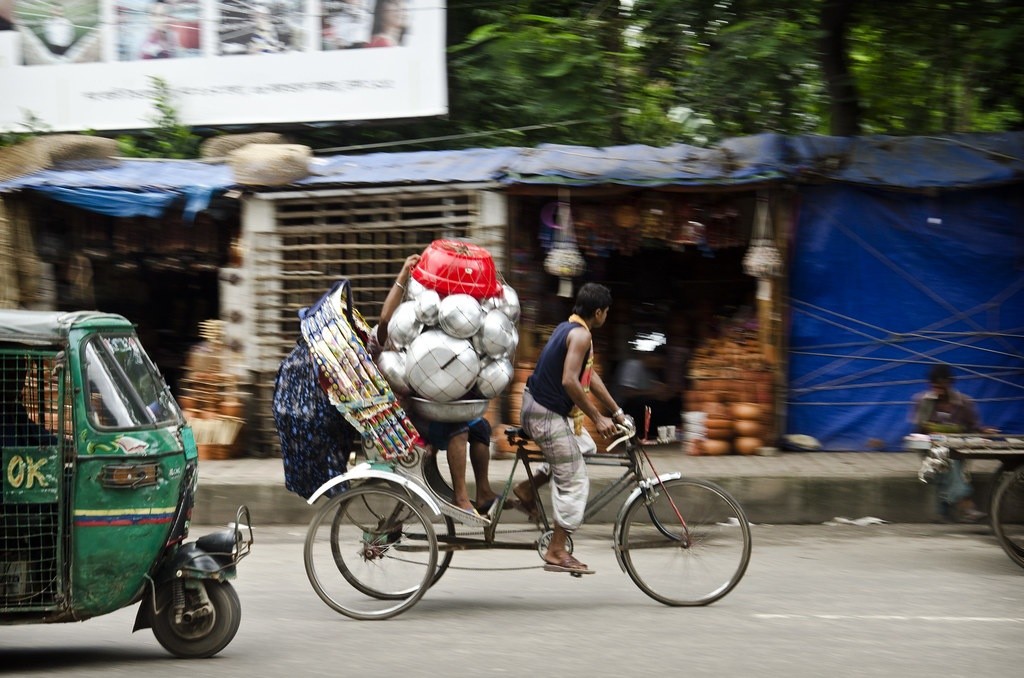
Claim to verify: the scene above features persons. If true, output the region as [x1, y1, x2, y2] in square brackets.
[0, 351, 75, 595]
[513, 283, 633, 573]
[369, 254, 519, 520]
[911, 364, 988, 523]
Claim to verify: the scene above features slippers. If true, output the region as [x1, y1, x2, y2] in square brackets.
[476, 495, 514, 514]
[466, 505, 480, 516]
[543, 555, 595, 574]
[507, 497, 546, 522]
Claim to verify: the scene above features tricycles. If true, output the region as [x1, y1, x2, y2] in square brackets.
[300, 277, 755, 621]
[924, 433, 1024, 575]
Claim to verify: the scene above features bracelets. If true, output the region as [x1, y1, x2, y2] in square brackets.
[612, 408, 623, 417]
[395, 281, 404, 288]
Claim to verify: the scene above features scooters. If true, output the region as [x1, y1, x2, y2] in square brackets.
[0, 307, 254, 660]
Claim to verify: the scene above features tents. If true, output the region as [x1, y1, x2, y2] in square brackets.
[0, 132, 1022, 453]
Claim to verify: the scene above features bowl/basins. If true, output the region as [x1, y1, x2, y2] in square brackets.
[411, 396, 490, 422]
[412, 240, 496, 301]
